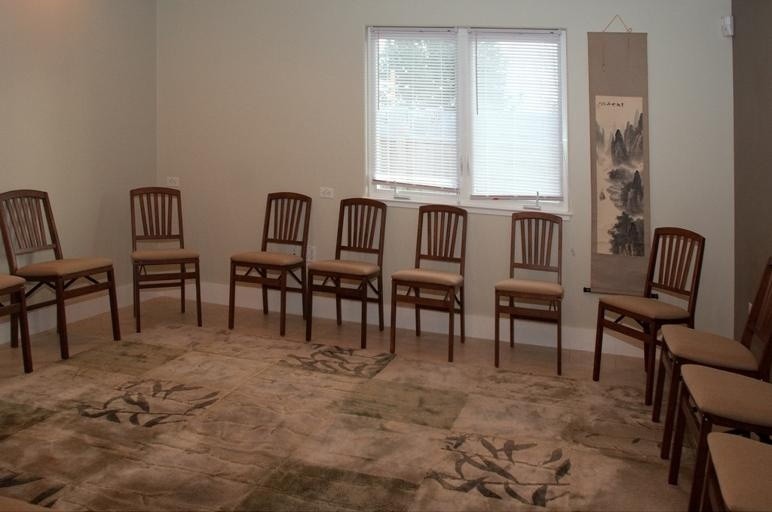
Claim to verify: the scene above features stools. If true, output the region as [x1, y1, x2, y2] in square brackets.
[665, 363, 771, 510]
[0, 274, 37, 373]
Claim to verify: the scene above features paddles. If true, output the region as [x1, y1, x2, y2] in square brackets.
[1, 319, 762, 512]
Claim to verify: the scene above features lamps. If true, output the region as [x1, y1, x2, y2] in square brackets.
[0, 187, 203, 360]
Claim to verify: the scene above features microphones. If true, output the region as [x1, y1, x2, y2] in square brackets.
[0, 274, 37, 373]
[665, 363, 771, 510]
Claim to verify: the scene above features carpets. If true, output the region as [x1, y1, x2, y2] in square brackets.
[1, 319, 762, 512]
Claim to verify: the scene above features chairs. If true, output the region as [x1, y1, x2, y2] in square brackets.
[0, 187, 203, 360]
[652, 254, 772, 467]
[389, 204, 469, 362]
[228, 190, 313, 336]
[494, 212, 563, 372]
[593, 225, 706, 405]
[306, 198, 388, 349]
